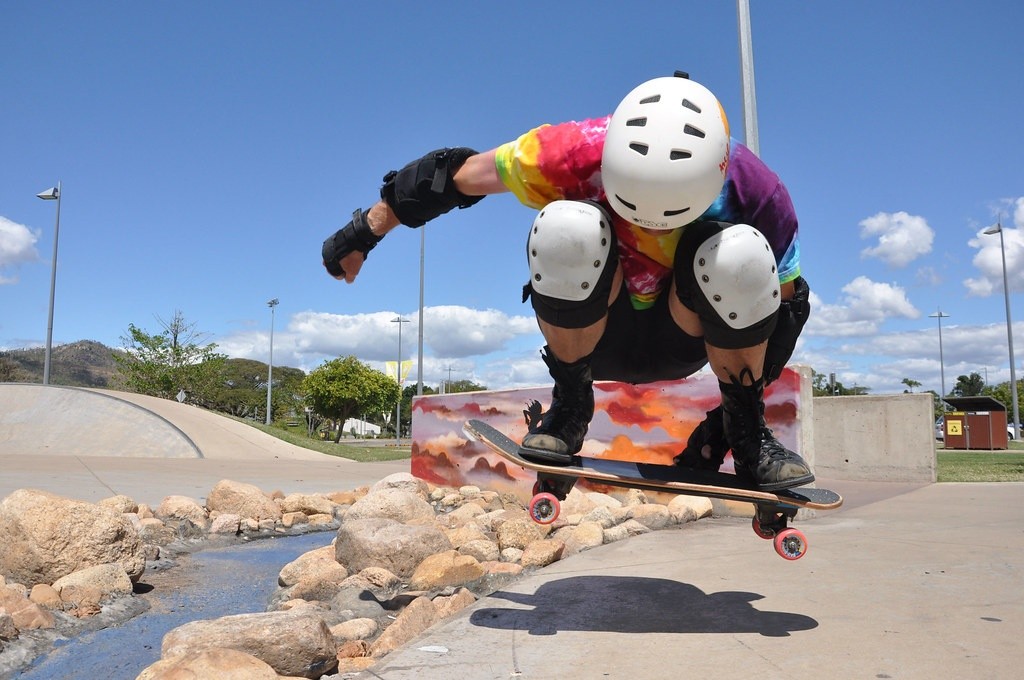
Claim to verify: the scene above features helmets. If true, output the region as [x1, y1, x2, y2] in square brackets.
[599, 75, 731, 230]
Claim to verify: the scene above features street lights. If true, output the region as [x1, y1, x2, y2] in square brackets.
[443, 364, 456, 393]
[983, 223, 1021, 440]
[36, 181, 61, 384]
[928, 305, 950, 412]
[264, 297, 280, 425]
[391, 314, 410, 446]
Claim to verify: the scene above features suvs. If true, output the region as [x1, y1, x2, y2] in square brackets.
[935, 415, 1015, 442]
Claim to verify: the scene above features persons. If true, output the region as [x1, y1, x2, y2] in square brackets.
[322, 71, 816, 492]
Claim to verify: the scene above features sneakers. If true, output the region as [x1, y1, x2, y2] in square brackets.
[717, 381, 815, 492]
[517, 345, 595, 467]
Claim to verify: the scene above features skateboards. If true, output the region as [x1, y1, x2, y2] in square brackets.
[462, 418, 845, 561]
